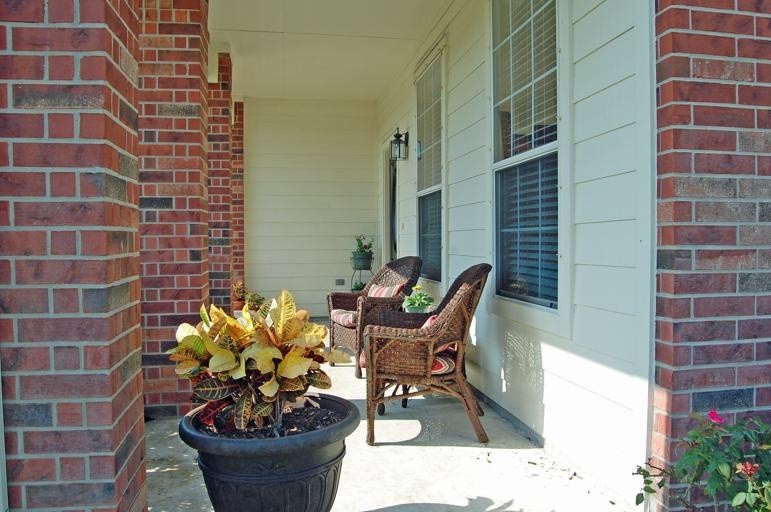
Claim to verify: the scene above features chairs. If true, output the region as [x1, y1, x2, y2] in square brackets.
[326, 255, 494, 445]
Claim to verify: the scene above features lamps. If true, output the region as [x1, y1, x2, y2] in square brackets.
[389, 126, 409, 161]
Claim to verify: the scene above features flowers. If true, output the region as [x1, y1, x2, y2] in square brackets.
[351, 231, 376, 252]
[632, 409, 771, 512]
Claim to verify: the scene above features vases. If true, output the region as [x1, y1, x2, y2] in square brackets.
[352, 251, 371, 269]
